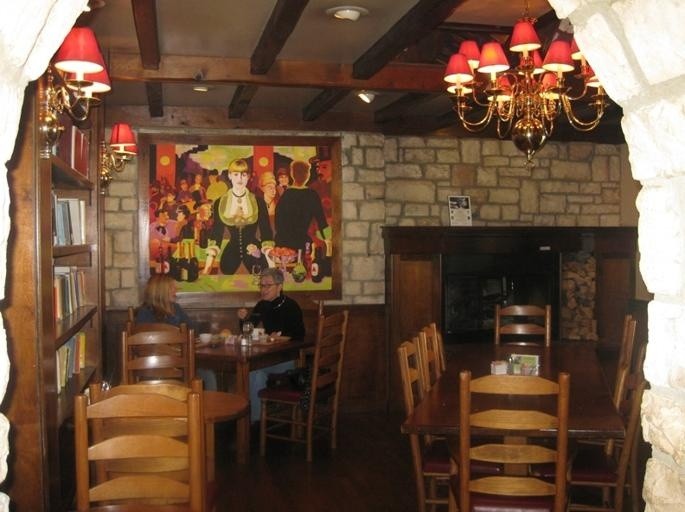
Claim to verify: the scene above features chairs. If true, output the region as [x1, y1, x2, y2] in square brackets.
[73, 382, 248, 512]
[121, 306, 196, 381]
[494, 304, 553, 343]
[258, 310, 349, 464]
[459, 371, 570, 512]
[568, 314, 647, 511]
[397, 324, 455, 511]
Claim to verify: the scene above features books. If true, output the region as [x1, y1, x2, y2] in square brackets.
[41, 108, 95, 396]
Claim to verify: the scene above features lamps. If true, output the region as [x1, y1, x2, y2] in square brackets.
[99, 123, 139, 197]
[39, 26, 112, 161]
[358, 93, 376, 103]
[135, 131, 343, 300]
[324, 5, 370, 21]
[446, 0, 611, 163]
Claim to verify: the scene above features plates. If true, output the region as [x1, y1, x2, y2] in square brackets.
[267, 255, 299, 263]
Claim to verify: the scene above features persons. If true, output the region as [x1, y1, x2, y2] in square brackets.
[135, 272, 223, 459]
[148, 144, 331, 283]
[237, 267, 306, 454]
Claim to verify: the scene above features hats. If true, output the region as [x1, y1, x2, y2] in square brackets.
[191, 188, 212, 211]
[313, 145, 330, 162]
[176, 204, 189, 213]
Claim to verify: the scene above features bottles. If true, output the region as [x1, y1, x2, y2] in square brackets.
[492, 357, 542, 376]
[155, 238, 201, 283]
[291, 240, 332, 283]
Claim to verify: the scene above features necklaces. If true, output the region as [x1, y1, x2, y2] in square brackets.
[269, 294, 287, 311]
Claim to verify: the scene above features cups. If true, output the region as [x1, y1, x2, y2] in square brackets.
[199, 322, 278, 347]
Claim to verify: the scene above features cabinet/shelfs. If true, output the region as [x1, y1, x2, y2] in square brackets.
[10, 81, 103, 512]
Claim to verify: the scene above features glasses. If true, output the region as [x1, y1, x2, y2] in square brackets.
[256, 283, 276, 288]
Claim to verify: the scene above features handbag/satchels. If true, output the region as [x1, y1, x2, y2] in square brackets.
[284, 366, 330, 390]
